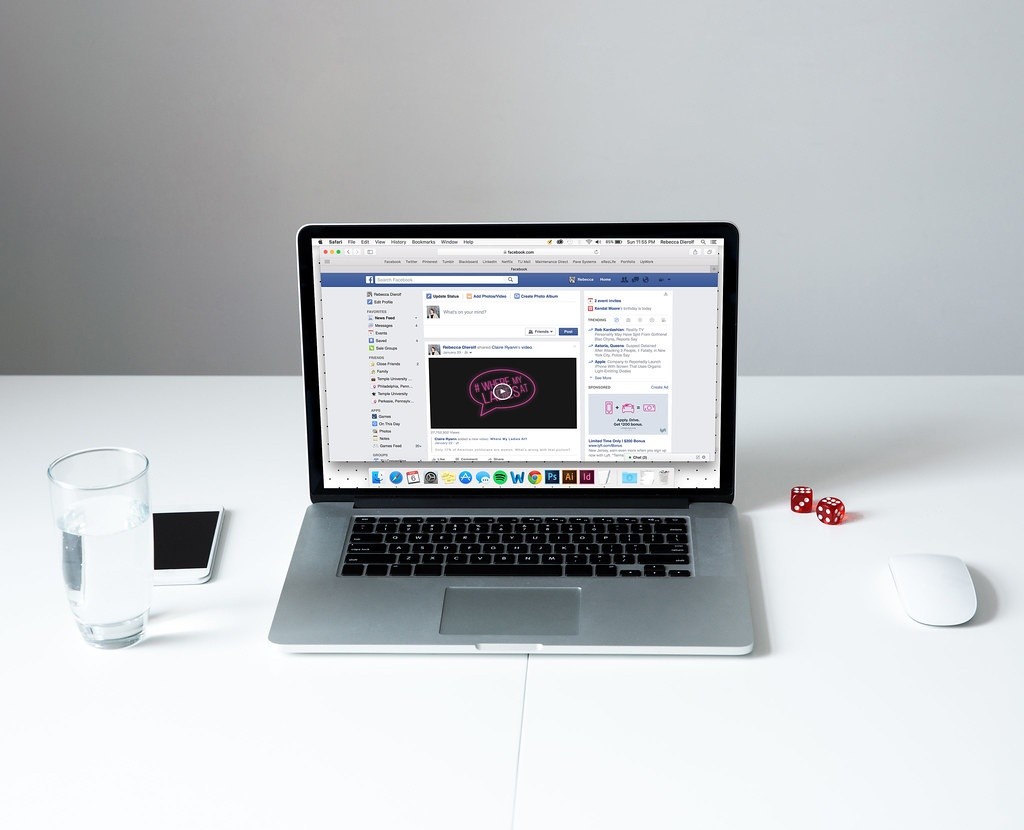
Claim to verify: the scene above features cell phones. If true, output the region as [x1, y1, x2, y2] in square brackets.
[152, 505, 224, 587]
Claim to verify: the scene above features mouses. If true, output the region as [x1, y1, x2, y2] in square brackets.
[888, 554, 978, 626]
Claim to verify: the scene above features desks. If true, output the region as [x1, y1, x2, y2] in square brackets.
[0, 375, 1024, 830]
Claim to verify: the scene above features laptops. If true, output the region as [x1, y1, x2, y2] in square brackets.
[267, 221, 755, 657]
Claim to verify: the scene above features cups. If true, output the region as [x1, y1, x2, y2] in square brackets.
[48, 446, 151, 650]
